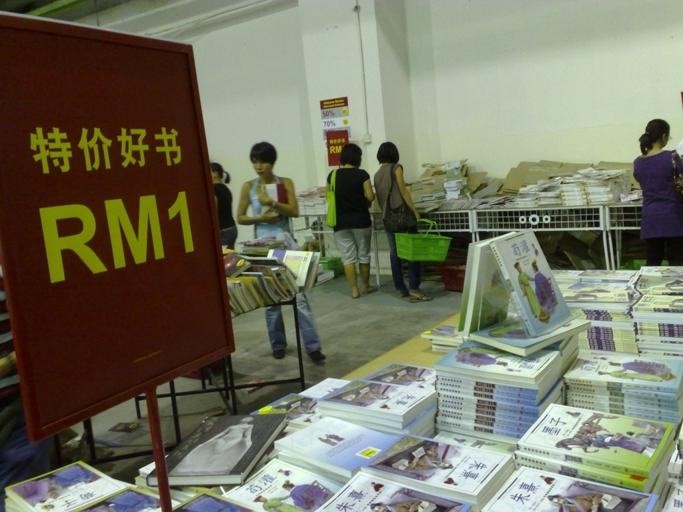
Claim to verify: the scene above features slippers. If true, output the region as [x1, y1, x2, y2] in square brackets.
[399, 287, 432, 302]
[351, 285, 378, 299]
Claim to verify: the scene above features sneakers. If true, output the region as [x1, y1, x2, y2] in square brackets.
[273, 346, 285, 359]
[311, 351, 326, 363]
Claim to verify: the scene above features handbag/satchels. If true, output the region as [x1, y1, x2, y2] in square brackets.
[325, 167, 339, 227]
[385, 198, 416, 234]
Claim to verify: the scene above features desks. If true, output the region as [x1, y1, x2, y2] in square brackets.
[53, 295, 306, 466]
[335, 308, 460, 383]
[291, 201, 645, 292]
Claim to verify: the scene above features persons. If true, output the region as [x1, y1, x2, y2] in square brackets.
[372, 143, 432, 303]
[209, 163, 237, 249]
[325, 142, 372, 299]
[633, 118, 682, 263]
[235, 142, 325, 363]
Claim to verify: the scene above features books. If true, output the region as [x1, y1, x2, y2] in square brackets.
[457, 231, 592, 355]
[275, 418, 682, 511]
[359, 362, 436, 391]
[223, 240, 335, 314]
[260, 183, 288, 220]
[423, 323, 683, 488]
[550, 265, 681, 356]
[298, 376, 438, 438]
[2, 391, 325, 511]
[295, 186, 326, 215]
[405, 159, 644, 214]
